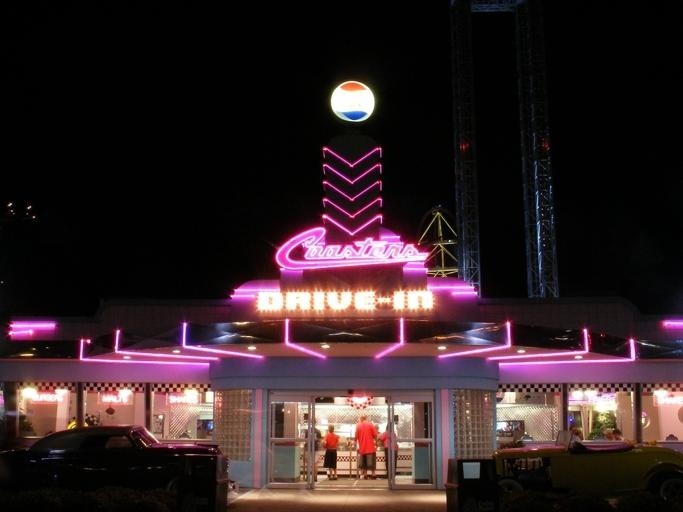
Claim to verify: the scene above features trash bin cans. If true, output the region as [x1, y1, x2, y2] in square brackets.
[444, 457, 500, 512]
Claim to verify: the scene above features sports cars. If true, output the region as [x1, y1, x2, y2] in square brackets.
[499, 426, 683, 502]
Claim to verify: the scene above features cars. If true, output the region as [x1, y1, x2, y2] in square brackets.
[0, 424, 223, 494]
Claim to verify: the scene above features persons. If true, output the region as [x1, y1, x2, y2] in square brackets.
[380, 422, 398, 478]
[324, 426, 339, 479]
[613, 429, 623, 441]
[573, 430, 584, 440]
[305, 419, 321, 481]
[602, 428, 615, 440]
[354, 415, 378, 480]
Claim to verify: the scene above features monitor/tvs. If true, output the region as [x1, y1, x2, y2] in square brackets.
[496, 421, 524, 437]
